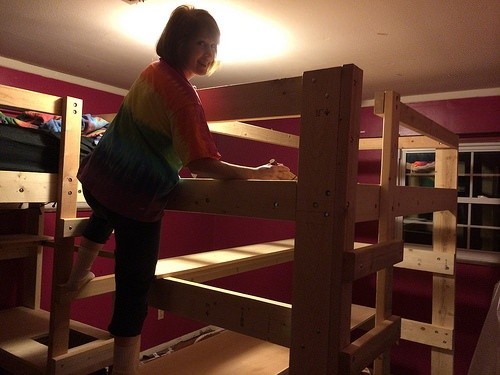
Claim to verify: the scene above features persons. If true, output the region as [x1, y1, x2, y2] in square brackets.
[65, 3, 294, 375]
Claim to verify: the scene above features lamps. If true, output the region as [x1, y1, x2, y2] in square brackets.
[105, 0, 297, 75]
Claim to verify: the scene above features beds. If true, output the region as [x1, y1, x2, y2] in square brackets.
[1, 62, 457, 375]
